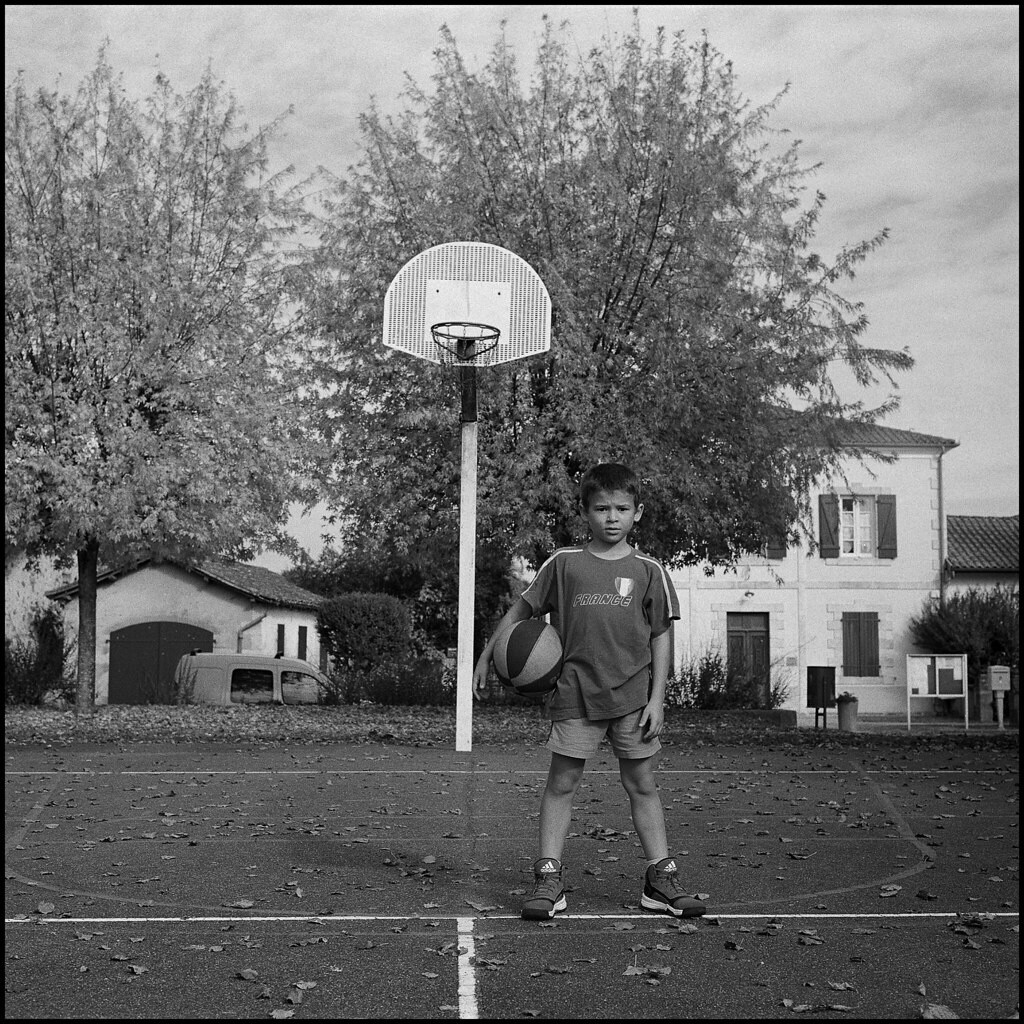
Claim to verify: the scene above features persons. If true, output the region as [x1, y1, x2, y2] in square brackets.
[473, 463, 707, 918]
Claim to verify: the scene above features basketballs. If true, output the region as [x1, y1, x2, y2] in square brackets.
[493, 618, 565, 696]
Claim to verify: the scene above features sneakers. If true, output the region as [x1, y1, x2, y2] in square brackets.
[522, 857, 567, 918]
[641, 856, 707, 916]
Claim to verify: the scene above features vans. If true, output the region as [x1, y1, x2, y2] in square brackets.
[175, 649, 364, 705]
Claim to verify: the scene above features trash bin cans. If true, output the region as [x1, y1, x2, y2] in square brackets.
[837, 696, 859, 733]
